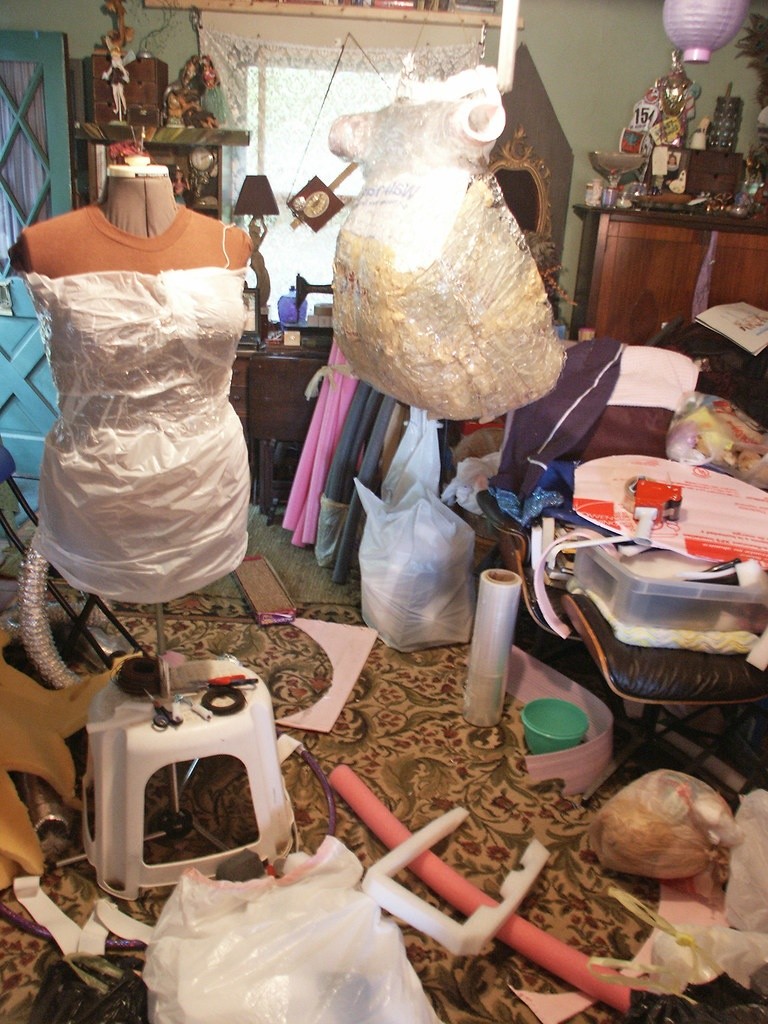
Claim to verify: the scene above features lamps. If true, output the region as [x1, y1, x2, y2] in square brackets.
[234, 174, 280, 306]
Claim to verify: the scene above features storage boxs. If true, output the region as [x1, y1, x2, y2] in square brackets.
[572, 528, 768, 632]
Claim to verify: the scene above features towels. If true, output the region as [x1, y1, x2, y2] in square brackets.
[486, 339, 625, 503]
[604, 344, 700, 414]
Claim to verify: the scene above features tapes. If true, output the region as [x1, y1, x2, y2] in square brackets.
[624, 475, 657, 512]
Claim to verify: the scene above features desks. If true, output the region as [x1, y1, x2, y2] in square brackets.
[246, 355, 329, 515]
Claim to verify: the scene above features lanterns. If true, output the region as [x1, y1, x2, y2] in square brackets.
[663, 0, 747, 64]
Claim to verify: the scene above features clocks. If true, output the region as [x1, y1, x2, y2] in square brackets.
[189, 146, 215, 184]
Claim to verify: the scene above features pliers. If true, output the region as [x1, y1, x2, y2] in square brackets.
[189, 675, 245, 685]
[169, 693, 213, 723]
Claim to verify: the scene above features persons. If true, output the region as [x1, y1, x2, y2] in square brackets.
[16, 162, 253, 600]
[667, 153, 679, 170]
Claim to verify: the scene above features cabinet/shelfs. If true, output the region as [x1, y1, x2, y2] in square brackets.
[91, 55, 169, 128]
[569, 203, 768, 344]
[73, 122, 250, 220]
[228, 350, 258, 440]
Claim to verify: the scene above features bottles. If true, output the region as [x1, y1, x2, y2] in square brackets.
[278, 286, 307, 330]
[585, 183, 593, 205]
[601, 187, 619, 209]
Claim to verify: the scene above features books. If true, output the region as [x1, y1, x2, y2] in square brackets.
[543, 520, 578, 589]
[695, 301, 768, 357]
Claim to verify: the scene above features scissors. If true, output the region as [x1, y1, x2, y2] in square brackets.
[143, 688, 182, 727]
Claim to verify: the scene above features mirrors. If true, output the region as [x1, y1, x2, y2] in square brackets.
[487, 123, 553, 238]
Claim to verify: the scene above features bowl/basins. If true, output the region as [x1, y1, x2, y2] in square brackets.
[519, 698, 590, 755]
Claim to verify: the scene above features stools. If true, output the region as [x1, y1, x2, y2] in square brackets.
[81, 661, 293, 901]
